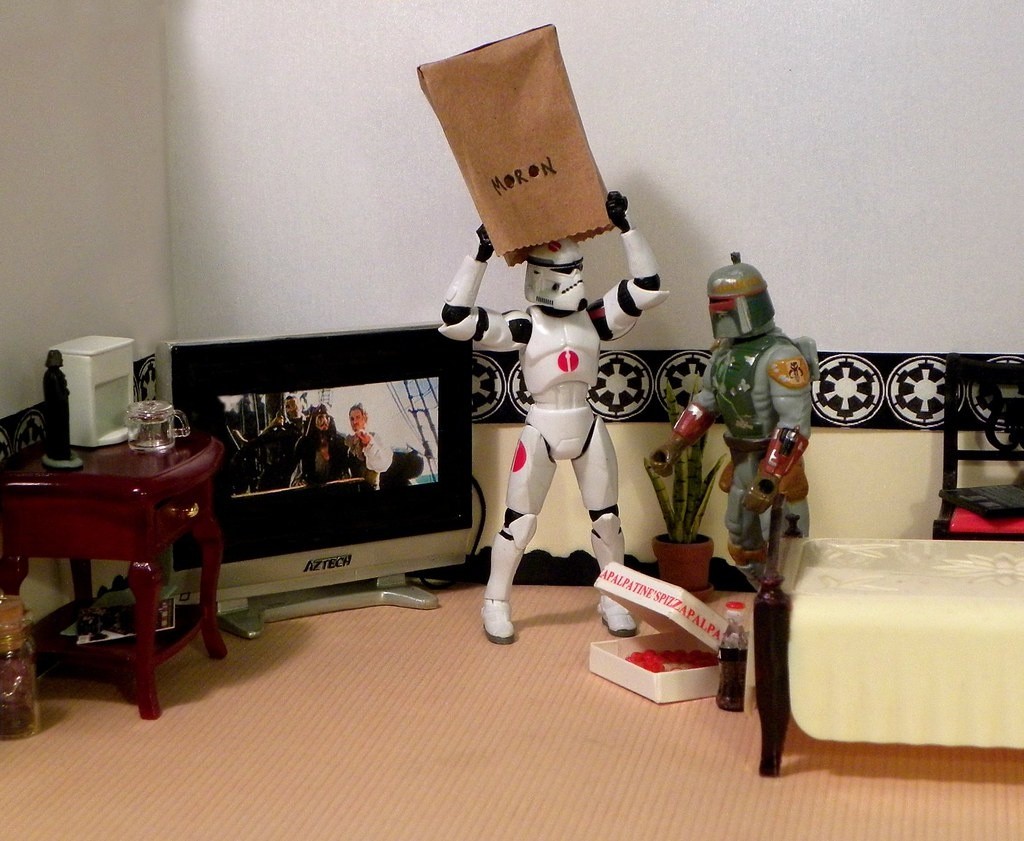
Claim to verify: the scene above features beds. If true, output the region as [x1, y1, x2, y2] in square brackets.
[755, 516, 1024, 778]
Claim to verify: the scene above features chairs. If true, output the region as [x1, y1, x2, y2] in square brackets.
[932, 354, 1024, 542]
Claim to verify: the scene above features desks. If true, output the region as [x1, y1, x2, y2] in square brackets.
[1, 429, 227, 719]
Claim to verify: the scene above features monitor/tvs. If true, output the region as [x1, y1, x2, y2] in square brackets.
[153, 320, 476, 603]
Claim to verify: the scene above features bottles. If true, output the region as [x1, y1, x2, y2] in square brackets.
[717, 602, 748, 709]
[0, 594, 41, 738]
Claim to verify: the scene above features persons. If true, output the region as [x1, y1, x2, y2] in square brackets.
[42, 349, 74, 462]
[647, 252, 814, 603]
[206, 392, 397, 501]
[434, 188, 669, 646]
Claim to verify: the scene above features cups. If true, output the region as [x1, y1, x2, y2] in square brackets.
[124, 400, 190, 453]
